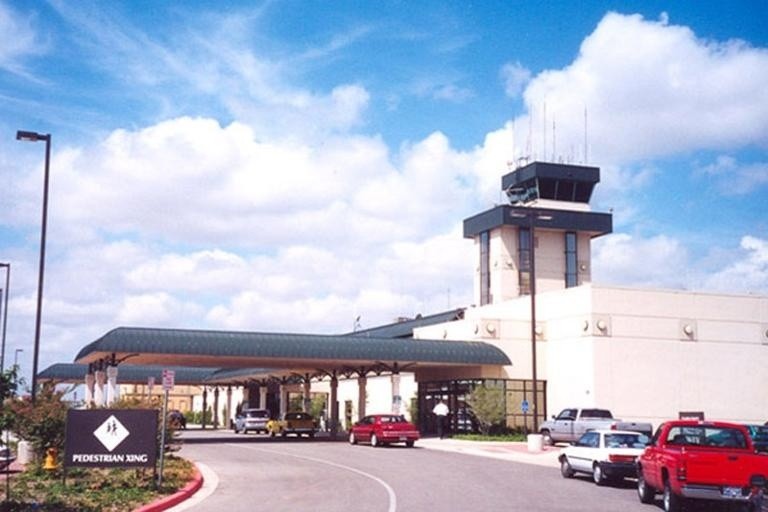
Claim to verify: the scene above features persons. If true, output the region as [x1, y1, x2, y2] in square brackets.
[433, 399, 451, 440]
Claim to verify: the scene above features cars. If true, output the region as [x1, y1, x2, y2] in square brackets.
[346, 414, 420, 447]
[266, 411, 319, 438]
[0, 438, 17, 470]
[234, 408, 271, 434]
[557, 430, 653, 485]
[706, 423, 768, 452]
[165, 409, 187, 429]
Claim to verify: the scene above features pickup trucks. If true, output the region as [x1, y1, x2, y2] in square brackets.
[538, 407, 653, 446]
[635, 420, 768, 512]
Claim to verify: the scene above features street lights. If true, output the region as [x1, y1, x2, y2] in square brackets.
[14, 349, 23, 396]
[0, 263, 11, 398]
[15, 130, 51, 441]
[509, 207, 553, 454]
